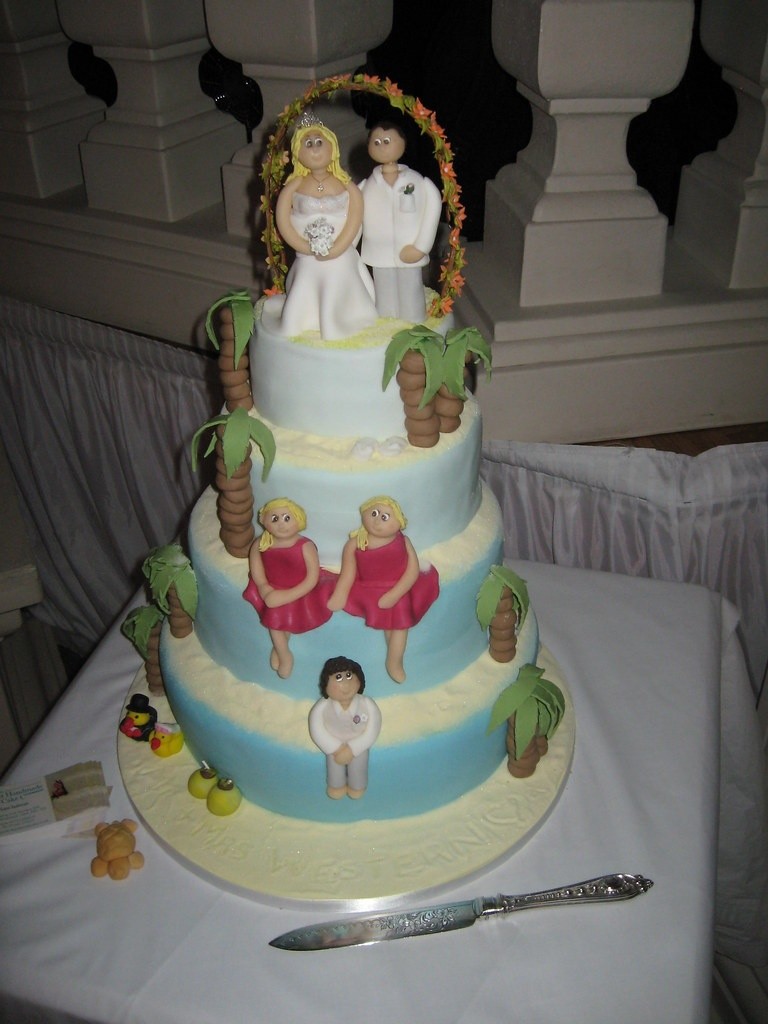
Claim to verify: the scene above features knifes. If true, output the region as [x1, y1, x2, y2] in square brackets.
[268, 873, 653, 951]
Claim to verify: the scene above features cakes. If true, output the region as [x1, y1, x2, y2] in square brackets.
[119, 109, 567, 822]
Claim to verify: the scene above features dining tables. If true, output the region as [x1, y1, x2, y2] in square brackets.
[2, 525, 768, 1023]
